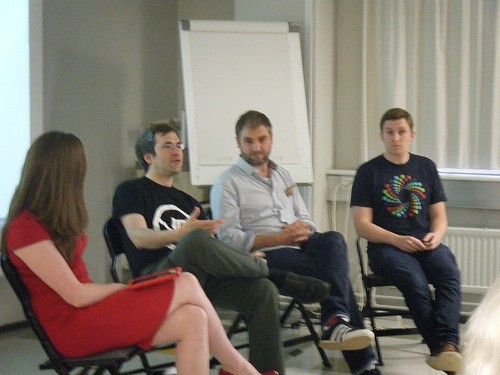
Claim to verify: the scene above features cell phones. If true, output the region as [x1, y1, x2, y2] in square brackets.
[128, 266, 182, 289]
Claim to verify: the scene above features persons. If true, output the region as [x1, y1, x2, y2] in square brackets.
[458, 280, 500, 375]
[350, 106, 464, 375]
[111, 118, 332, 375]
[209, 111, 382, 375]
[1, 131, 279, 375]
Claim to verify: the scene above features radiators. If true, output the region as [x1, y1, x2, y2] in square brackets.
[441, 228, 500, 293]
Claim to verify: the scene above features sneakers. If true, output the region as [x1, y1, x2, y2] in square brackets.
[427, 342, 463, 373]
[318, 317, 375, 351]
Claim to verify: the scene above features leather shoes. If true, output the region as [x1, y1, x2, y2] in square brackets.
[280, 272, 331, 301]
[219, 366, 278, 375]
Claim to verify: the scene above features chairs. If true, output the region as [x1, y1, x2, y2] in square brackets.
[0, 252, 152, 375]
[356, 236, 428, 367]
[103, 216, 177, 375]
[200, 202, 334, 370]
